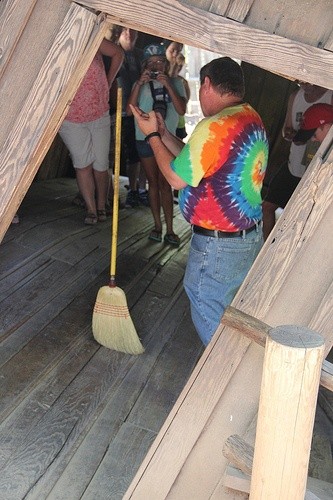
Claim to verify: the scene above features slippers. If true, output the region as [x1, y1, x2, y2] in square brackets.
[165, 233, 181, 243]
[148, 229, 162, 241]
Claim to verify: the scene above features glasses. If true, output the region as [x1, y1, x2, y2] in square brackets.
[146, 59, 165, 66]
[296, 82, 313, 87]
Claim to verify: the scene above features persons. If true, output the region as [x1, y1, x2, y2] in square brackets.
[56, 26, 190, 244]
[259, 77, 333, 228]
[127, 57, 269, 350]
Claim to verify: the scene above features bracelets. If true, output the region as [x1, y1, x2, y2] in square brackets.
[144, 131, 161, 143]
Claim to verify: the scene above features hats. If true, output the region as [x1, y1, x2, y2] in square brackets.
[293, 103, 333, 146]
[143, 44, 165, 57]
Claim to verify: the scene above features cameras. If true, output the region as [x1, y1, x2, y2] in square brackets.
[148, 71, 159, 79]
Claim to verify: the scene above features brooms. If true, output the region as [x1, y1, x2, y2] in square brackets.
[90, 87, 146, 356]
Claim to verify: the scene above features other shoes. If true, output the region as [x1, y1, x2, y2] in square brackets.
[72, 195, 85, 208]
[137, 188, 150, 207]
[83, 213, 97, 225]
[125, 191, 137, 208]
[97, 210, 107, 221]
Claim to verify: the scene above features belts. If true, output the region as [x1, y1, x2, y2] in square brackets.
[190, 220, 261, 238]
